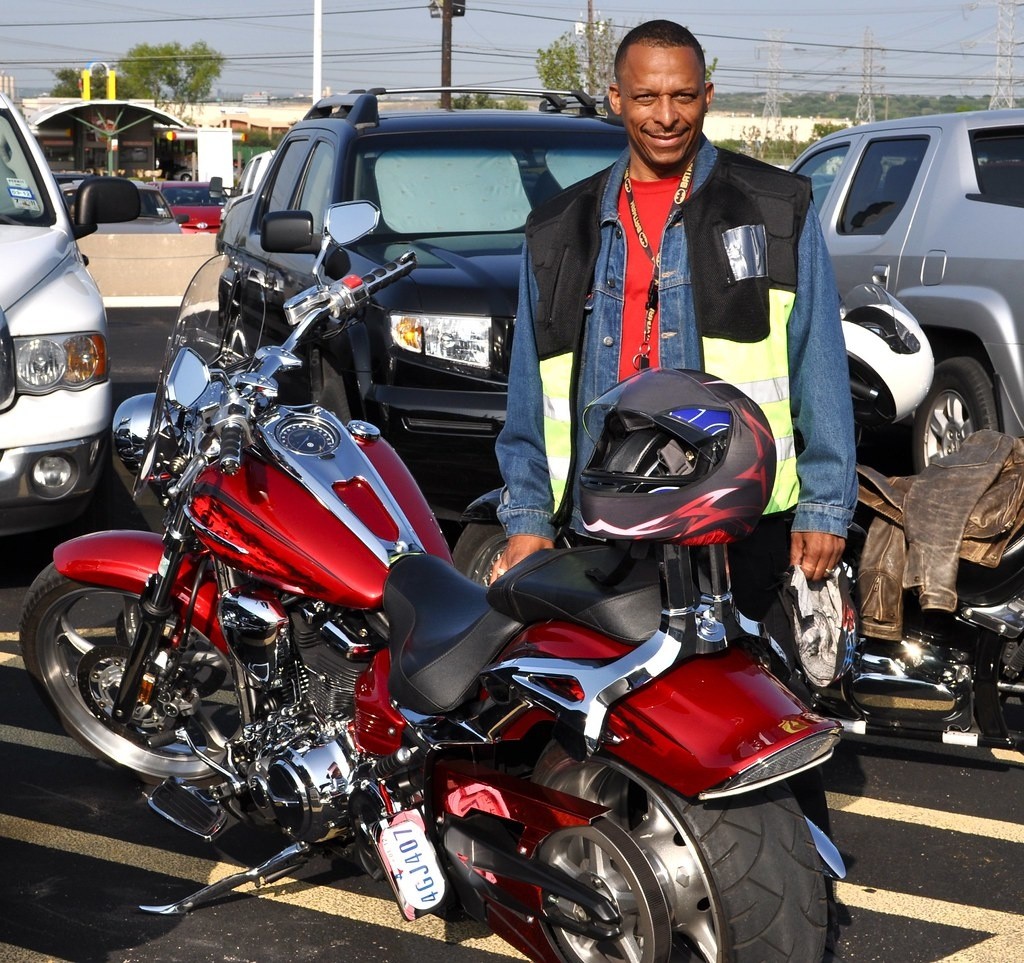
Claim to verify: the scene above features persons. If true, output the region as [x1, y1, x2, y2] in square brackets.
[489, 21, 856, 835]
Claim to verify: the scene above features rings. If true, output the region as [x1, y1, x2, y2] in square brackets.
[824, 568, 835, 576]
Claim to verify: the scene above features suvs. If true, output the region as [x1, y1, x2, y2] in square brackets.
[216, 81, 633, 552]
[778, 110, 1024, 474]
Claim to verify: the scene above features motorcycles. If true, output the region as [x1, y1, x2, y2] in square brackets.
[725, 356, 1024, 760]
[12, 193, 871, 962]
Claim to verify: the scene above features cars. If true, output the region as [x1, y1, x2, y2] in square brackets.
[151, 180, 228, 238]
[52, 168, 190, 236]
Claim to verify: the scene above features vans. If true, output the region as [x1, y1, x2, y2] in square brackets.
[207, 148, 283, 220]
[0, 91, 143, 574]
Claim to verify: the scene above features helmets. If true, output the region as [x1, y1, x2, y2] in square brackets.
[579, 369, 779, 545]
[839, 304, 935, 424]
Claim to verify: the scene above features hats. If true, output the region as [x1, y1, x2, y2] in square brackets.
[780, 557, 858, 687]
